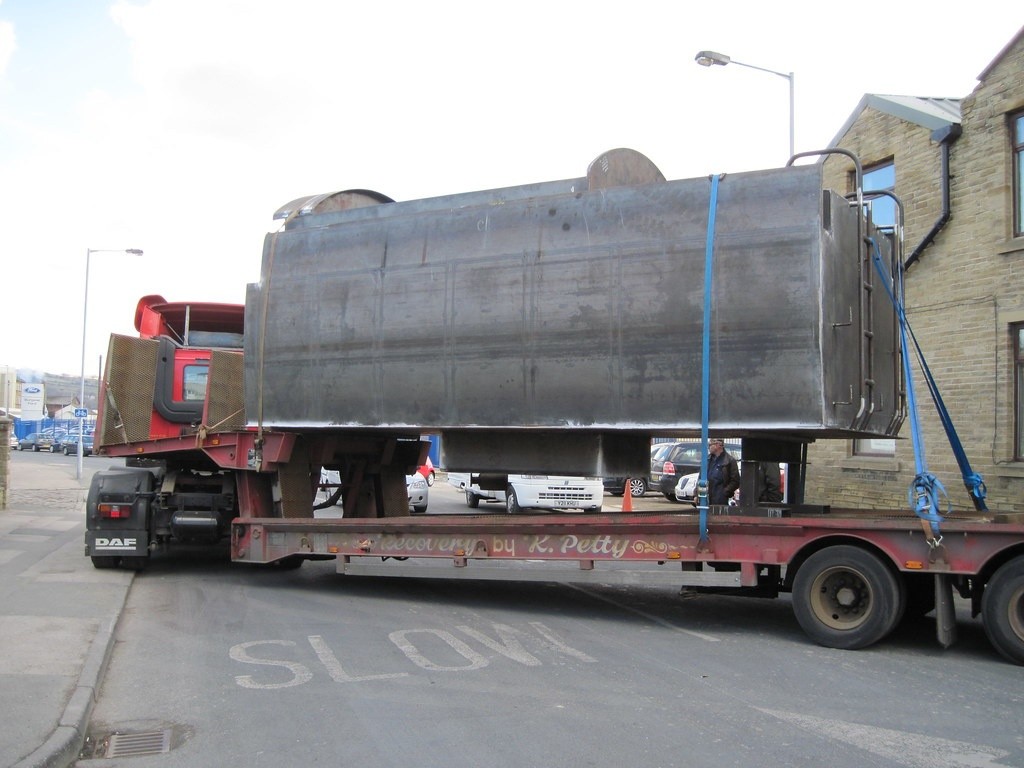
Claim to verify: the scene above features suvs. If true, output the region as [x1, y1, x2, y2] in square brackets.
[603, 440, 669, 498]
[649, 442, 742, 502]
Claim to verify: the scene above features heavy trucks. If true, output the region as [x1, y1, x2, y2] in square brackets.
[81, 144, 1024, 674]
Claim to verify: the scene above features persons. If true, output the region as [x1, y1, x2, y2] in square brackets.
[758, 461, 783, 503]
[693, 438, 740, 506]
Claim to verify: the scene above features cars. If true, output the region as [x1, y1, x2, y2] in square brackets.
[415, 454, 436, 487]
[10, 432, 19, 450]
[18, 433, 55, 452]
[673, 460, 787, 507]
[41, 423, 96, 453]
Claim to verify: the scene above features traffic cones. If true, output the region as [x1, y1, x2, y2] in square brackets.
[620, 476, 633, 514]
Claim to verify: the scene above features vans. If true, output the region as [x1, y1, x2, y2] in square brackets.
[61, 434, 94, 457]
[447, 471, 604, 514]
[318, 466, 428, 513]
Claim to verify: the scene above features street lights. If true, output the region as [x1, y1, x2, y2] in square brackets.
[75, 248, 143, 482]
[696, 50, 795, 160]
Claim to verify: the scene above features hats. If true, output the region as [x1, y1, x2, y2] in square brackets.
[710, 438, 724, 444]
